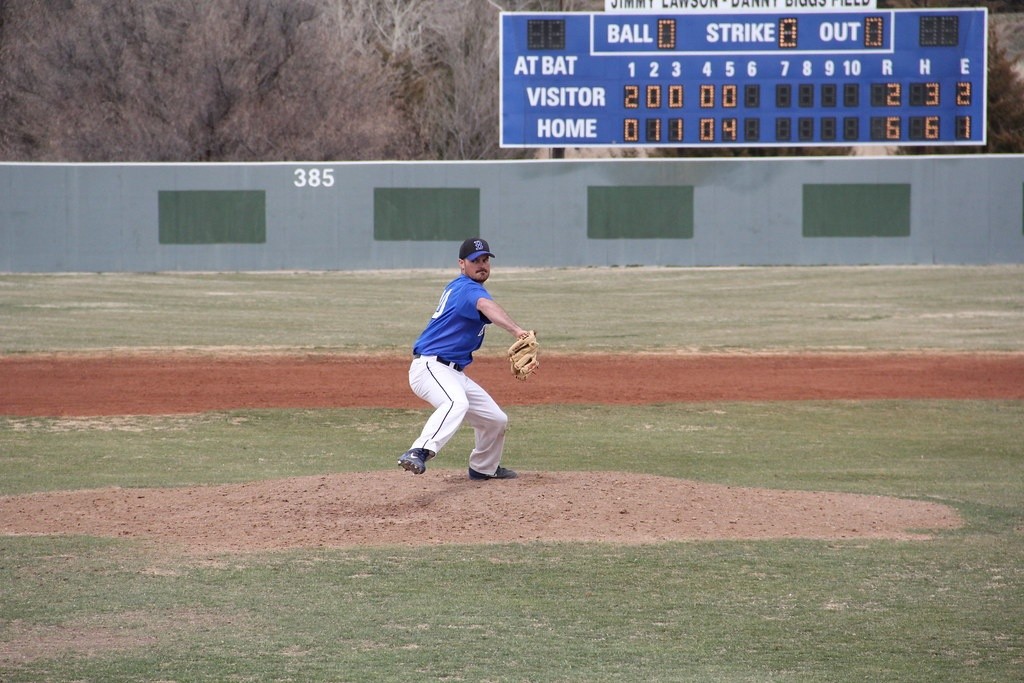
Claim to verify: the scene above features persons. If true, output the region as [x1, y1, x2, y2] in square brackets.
[397, 237, 540, 480]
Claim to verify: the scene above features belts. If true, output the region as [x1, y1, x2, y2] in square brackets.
[413, 353, 463, 372]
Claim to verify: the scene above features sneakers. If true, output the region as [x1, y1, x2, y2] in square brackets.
[468, 466, 517, 481]
[397, 448, 428, 475]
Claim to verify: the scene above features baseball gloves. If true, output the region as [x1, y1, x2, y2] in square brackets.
[506, 329, 541, 382]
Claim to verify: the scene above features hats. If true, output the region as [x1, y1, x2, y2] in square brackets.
[459, 238, 496, 261]
